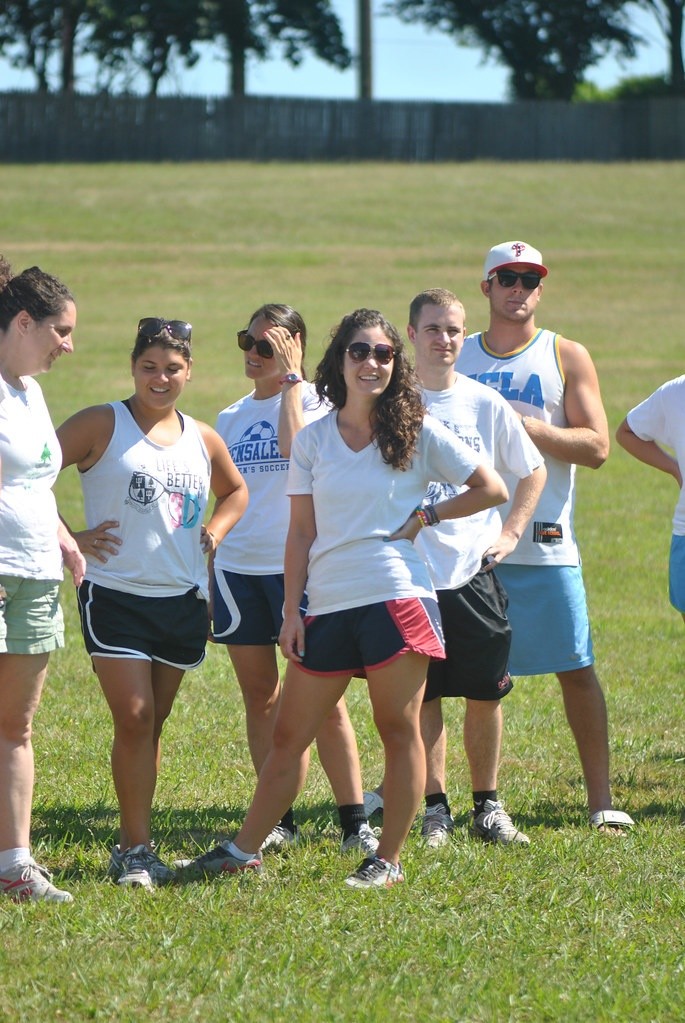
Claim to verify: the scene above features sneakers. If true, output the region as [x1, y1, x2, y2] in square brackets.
[260, 823, 301, 853]
[0, 859, 74, 906]
[106, 843, 186, 890]
[467, 799, 530, 847]
[342, 853, 407, 890]
[339, 820, 381, 857]
[419, 802, 455, 852]
[171, 838, 263, 881]
[360, 789, 386, 821]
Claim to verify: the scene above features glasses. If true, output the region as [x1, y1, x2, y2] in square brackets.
[236, 328, 275, 359]
[136, 317, 193, 345]
[487, 269, 541, 290]
[342, 341, 399, 365]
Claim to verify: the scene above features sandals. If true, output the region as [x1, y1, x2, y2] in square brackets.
[588, 810, 634, 841]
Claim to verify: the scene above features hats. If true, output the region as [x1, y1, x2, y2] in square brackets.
[483, 240, 548, 281]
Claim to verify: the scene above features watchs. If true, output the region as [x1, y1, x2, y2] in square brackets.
[278, 373, 302, 386]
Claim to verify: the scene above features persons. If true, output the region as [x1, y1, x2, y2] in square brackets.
[209, 304, 381, 858]
[188, 309, 509, 886]
[401, 287, 548, 850]
[0, 266, 87, 906]
[363, 241, 636, 842]
[55, 321, 250, 890]
[616, 372, 685, 624]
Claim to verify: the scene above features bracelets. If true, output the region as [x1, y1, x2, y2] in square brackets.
[420, 507, 440, 527]
